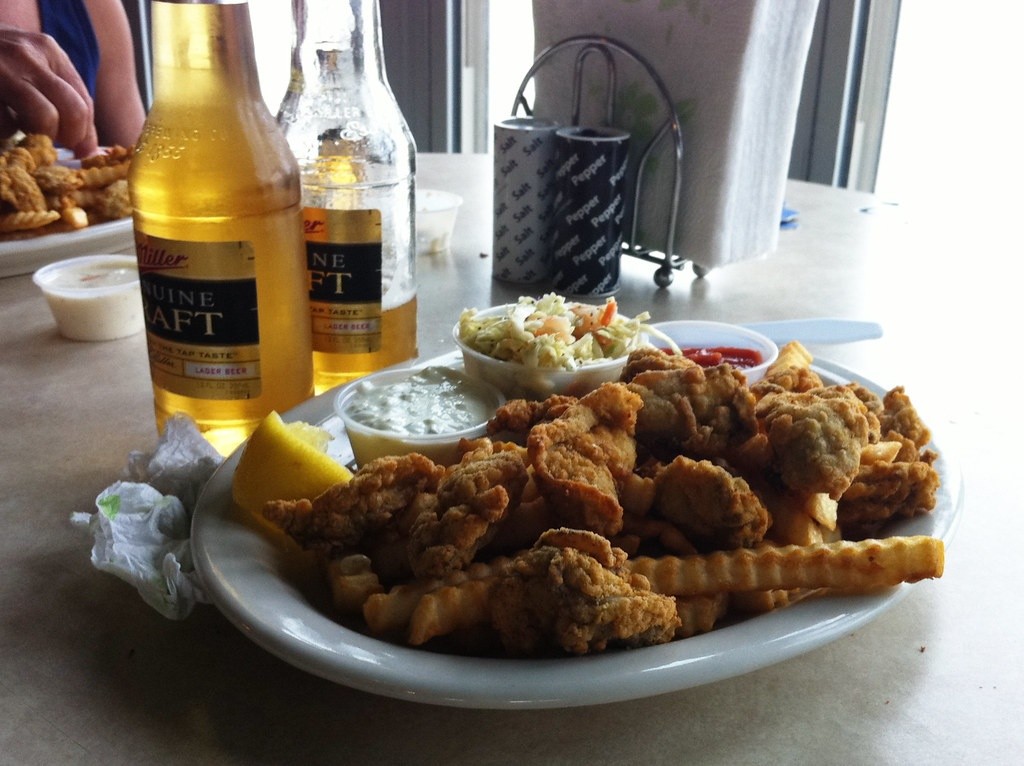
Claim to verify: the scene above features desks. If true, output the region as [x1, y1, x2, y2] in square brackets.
[0, 153, 1024, 766]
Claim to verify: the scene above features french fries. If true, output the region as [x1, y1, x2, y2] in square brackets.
[0, 159, 129, 242]
[333, 485, 949, 645]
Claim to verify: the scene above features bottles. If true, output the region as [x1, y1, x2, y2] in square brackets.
[127, 0, 314, 460]
[276, 0, 418, 396]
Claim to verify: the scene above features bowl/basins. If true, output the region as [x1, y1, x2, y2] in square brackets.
[451, 303, 638, 403]
[644, 320, 780, 389]
[33, 253, 145, 341]
[333, 366, 507, 472]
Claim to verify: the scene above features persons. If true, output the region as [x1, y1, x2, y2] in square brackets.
[0, 0, 146, 161]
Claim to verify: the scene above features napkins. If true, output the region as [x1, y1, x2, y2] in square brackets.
[531, 0, 819, 267]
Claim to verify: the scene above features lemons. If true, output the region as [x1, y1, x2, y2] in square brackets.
[233, 410, 355, 533]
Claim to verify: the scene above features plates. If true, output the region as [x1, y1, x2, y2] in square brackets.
[0, 148, 137, 278]
[189, 348, 964, 711]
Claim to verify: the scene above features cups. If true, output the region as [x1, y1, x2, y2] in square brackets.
[492, 117, 560, 284]
[556, 125, 633, 301]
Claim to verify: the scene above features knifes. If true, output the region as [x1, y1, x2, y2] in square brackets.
[733, 320, 883, 345]
